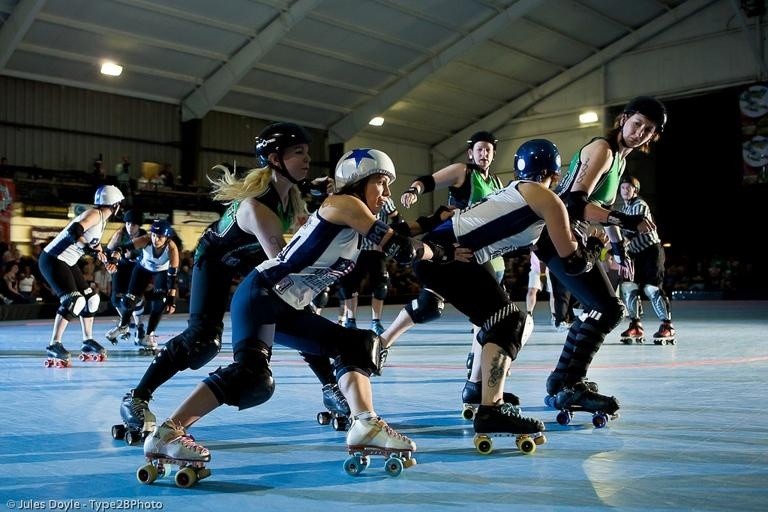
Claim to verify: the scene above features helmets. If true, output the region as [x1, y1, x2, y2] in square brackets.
[623, 97, 667, 133]
[467, 131, 497, 151]
[94, 185, 125, 207]
[150, 221, 173, 236]
[124, 211, 145, 226]
[514, 140, 562, 179]
[620, 176, 641, 190]
[255, 122, 312, 167]
[335, 148, 396, 193]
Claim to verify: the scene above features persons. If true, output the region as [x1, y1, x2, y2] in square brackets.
[39, 185, 125, 368]
[372, 138, 610, 455]
[372, 134, 506, 380]
[534, 98, 667, 428]
[619, 178, 676, 347]
[1, 261, 22, 301]
[136, 147, 472, 489]
[341, 198, 404, 336]
[105, 211, 148, 328]
[11, 248, 20, 267]
[18, 267, 39, 298]
[106, 220, 179, 356]
[1, 244, 14, 265]
[110, 122, 351, 446]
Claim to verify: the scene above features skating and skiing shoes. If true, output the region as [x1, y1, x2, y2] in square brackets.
[135, 324, 144, 345]
[461, 381, 520, 420]
[139, 332, 157, 355]
[380, 344, 387, 374]
[346, 321, 355, 328]
[555, 381, 622, 428]
[545, 372, 599, 408]
[371, 323, 385, 335]
[653, 328, 678, 345]
[467, 353, 474, 377]
[473, 405, 546, 454]
[136, 418, 212, 488]
[106, 324, 131, 345]
[79, 339, 107, 362]
[112, 389, 155, 446]
[42, 343, 71, 367]
[620, 320, 647, 344]
[317, 383, 353, 431]
[342, 412, 418, 477]
